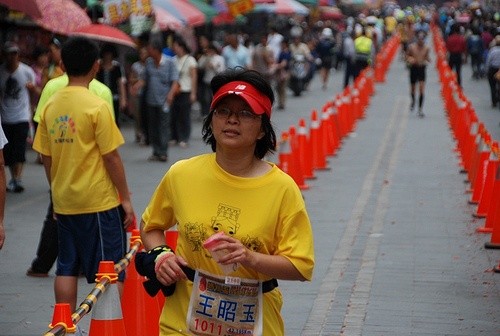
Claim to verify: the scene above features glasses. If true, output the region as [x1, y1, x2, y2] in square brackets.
[213, 106, 263, 123]
[52, 37, 62, 48]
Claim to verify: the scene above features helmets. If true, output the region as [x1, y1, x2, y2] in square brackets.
[291, 28, 303, 37]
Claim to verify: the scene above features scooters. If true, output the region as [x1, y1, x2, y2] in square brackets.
[287, 52, 310, 97]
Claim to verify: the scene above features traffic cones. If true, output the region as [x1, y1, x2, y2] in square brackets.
[276, 35, 399, 190]
[49, 303, 78, 336]
[89, 261, 126, 336]
[120, 228, 178, 336]
[428, 20, 500, 249]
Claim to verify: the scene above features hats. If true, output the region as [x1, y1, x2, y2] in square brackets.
[211, 80, 272, 120]
[322, 28, 331, 36]
[4, 41, 19, 52]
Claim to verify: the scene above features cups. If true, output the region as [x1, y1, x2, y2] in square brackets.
[202, 231, 237, 276]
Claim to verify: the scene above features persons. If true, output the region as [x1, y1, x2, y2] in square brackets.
[134, 66, 314, 336]
[403, 29, 431, 115]
[394, 0, 500, 90]
[0, 120, 8, 249]
[32, 38, 134, 313]
[487, 37, 500, 107]
[0, 12, 402, 190]
[25, 71, 114, 278]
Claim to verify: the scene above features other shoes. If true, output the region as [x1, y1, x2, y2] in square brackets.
[27, 268, 48, 277]
[6, 175, 25, 193]
[61, 325, 88, 336]
[418, 110, 424, 116]
[168, 140, 187, 148]
[149, 155, 167, 162]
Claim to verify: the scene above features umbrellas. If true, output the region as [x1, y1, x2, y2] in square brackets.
[0, 0, 390, 49]
